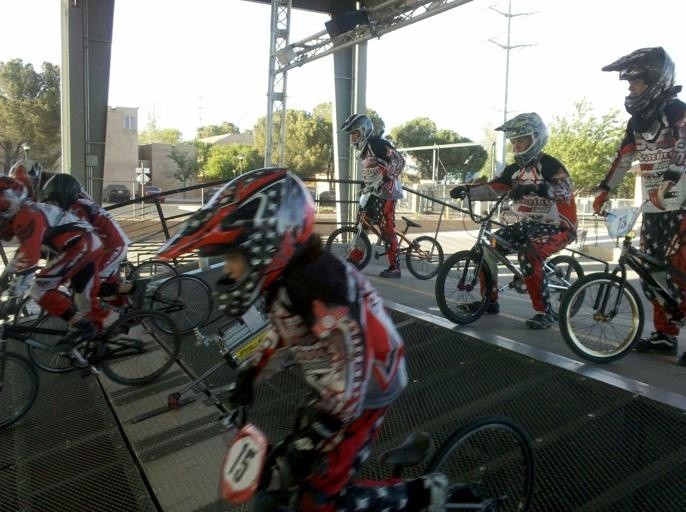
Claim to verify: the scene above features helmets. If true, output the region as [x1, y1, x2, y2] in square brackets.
[336, 114, 375, 152]
[0, 159, 82, 243]
[155, 166, 317, 319]
[601, 47, 677, 116]
[494, 113, 549, 169]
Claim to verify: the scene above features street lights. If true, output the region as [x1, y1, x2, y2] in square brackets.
[20, 142, 30, 159]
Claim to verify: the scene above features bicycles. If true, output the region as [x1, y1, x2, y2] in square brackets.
[0, 301, 184, 432]
[432, 181, 587, 328]
[221, 395, 536, 512]
[557, 191, 686, 365]
[23, 272, 216, 376]
[323, 180, 446, 282]
[11, 258, 182, 338]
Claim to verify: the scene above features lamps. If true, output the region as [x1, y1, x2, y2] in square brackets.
[275, 46, 307, 68]
[325, 8, 383, 47]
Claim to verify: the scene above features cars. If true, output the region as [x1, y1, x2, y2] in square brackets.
[321, 190, 337, 207]
[134, 185, 166, 204]
[102, 183, 132, 204]
[202, 187, 221, 204]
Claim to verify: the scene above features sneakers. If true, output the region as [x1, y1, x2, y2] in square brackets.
[465, 298, 501, 315]
[47, 318, 98, 356]
[380, 266, 401, 278]
[404, 472, 450, 512]
[633, 330, 679, 356]
[525, 301, 555, 329]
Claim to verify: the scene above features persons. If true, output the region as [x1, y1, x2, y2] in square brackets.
[1, 175, 106, 355]
[337, 113, 405, 277]
[40, 172, 130, 323]
[594, 47, 685, 365]
[156, 169, 409, 512]
[451, 112, 578, 328]
[10, 160, 91, 207]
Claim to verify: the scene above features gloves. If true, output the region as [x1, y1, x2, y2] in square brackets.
[593, 194, 610, 215]
[271, 409, 348, 492]
[649, 181, 673, 210]
[449, 185, 470, 200]
[510, 183, 539, 201]
[227, 365, 257, 411]
